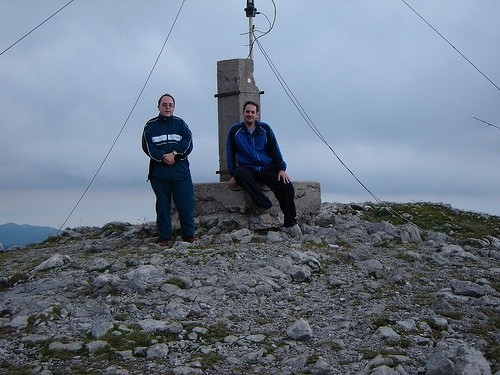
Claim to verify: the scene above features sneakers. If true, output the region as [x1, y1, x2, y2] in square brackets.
[253, 205, 273, 216]
[282, 223, 303, 237]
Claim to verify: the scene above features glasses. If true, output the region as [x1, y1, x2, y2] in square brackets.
[160, 103, 175, 107]
[244, 110, 257, 114]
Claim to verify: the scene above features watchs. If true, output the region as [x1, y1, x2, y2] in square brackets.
[172, 150, 177, 156]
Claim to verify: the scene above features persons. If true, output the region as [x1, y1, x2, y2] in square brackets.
[142, 94, 195, 245]
[227, 101, 302, 236]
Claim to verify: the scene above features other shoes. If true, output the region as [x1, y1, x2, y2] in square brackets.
[159, 240, 171, 246]
[184, 236, 199, 243]
